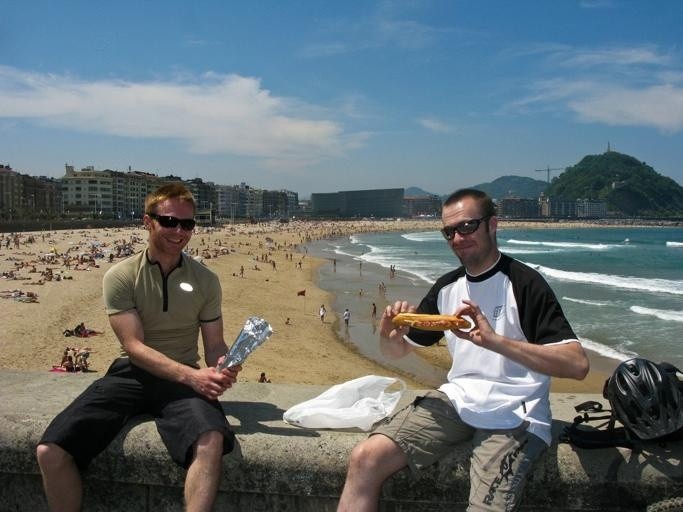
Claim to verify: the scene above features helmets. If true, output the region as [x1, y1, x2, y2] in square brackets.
[603, 358, 682, 441]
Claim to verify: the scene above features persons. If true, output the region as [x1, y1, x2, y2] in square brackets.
[333, 187, 591, 512]
[33, 182, 244, 512]
[58, 346, 90, 372]
[370, 302, 376, 320]
[359, 261, 361, 268]
[318, 304, 326, 321]
[79, 322, 88, 337]
[284, 318, 290, 324]
[359, 289, 362, 296]
[73, 325, 81, 337]
[334, 215, 443, 244]
[257, 372, 270, 382]
[378, 281, 387, 296]
[342, 308, 350, 325]
[389, 264, 396, 277]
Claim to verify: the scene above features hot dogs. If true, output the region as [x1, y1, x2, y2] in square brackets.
[391, 312, 471, 331]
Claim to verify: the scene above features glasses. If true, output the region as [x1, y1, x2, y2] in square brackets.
[150, 213, 196, 231]
[440, 219, 485, 239]
